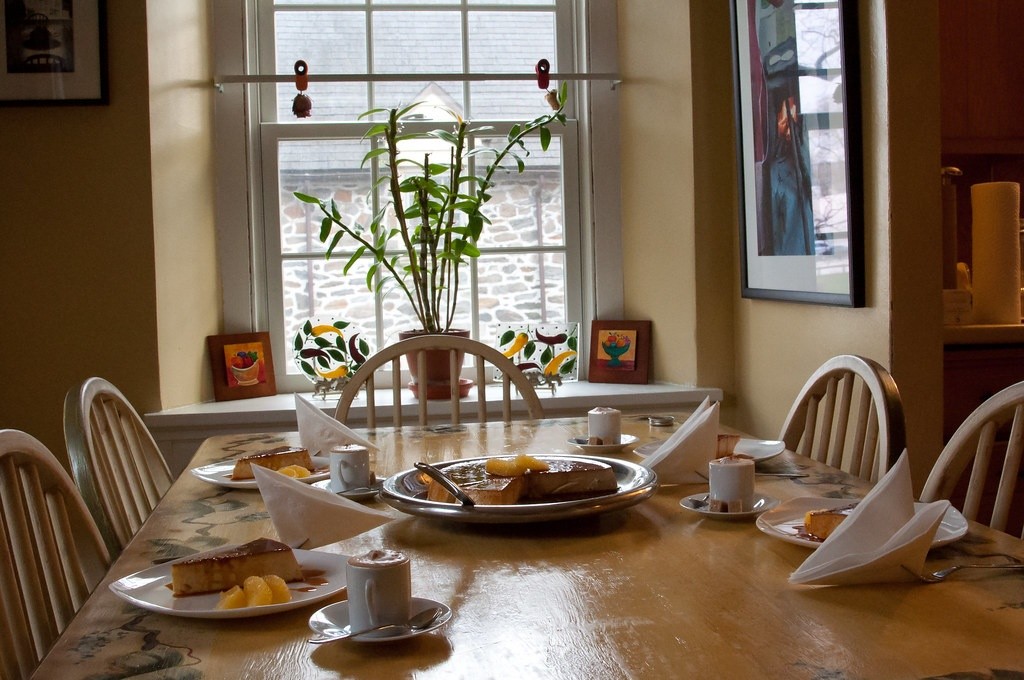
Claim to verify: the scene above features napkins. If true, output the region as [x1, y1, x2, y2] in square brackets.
[293, 392, 381, 457]
[639, 394, 721, 485]
[249, 462, 398, 550]
[786, 448, 951, 585]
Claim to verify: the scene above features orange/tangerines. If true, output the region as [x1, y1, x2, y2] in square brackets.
[216, 575, 292, 610]
[279, 464, 311, 478]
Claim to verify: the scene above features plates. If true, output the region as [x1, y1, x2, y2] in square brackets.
[308, 597, 452, 642]
[106, 541, 352, 618]
[632, 434, 785, 465]
[679, 492, 780, 517]
[309, 475, 386, 499]
[755, 496, 969, 550]
[378, 453, 657, 523]
[565, 432, 640, 452]
[191, 456, 332, 488]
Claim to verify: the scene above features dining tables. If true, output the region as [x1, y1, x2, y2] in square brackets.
[30, 408, 1024, 680]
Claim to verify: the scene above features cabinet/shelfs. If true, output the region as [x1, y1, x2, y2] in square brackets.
[938, 1, 1024, 537]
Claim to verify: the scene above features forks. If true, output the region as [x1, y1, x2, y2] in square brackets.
[900, 561, 1024, 582]
[694, 470, 811, 482]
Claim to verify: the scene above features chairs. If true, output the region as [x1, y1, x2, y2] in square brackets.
[63, 376, 174, 555]
[777, 353, 906, 484]
[0, 428, 112, 679]
[334, 334, 546, 429]
[919, 380, 1024, 542]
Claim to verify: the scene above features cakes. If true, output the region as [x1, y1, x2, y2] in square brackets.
[232, 446, 315, 479]
[717, 433, 741, 458]
[806, 501, 861, 539]
[426, 453, 618, 505]
[172, 536, 303, 596]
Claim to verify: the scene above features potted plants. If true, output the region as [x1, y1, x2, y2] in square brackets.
[292, 79, 567, 400]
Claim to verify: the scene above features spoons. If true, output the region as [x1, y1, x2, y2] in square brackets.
[307, 607, 443, 645]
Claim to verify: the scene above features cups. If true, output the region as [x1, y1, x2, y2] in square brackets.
[346, 548, 412, 637]
[588, 407, 621, 445]
[329, 445, 371, 492]
[707, 457, 756, 514]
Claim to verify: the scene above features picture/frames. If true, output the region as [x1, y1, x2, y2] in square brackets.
[588, 319, 652, 385]
[730, 0, 864, 308]
[0, 0, 110, 107]
[208, 331, 276, 402]
